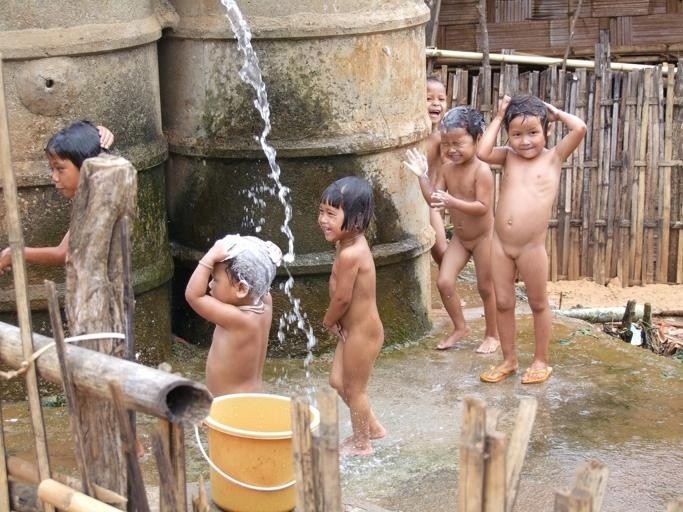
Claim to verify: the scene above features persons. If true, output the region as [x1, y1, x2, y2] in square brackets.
[184, 234, 284, 398]
[0, 118, 144, 459]
[317, 176, 385, 457]
[403, 106, 500, 353]
[476, 94, 587, 384]
[426, 78, 448, 270]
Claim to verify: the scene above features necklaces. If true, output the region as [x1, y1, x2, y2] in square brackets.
[237, 302, 267, 314]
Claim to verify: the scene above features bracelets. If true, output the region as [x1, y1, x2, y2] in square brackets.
[199, 259, 214, 271]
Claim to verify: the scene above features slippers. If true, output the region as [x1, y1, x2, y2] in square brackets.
[480, 365, 516, 383]
[521, 366, 553, 384]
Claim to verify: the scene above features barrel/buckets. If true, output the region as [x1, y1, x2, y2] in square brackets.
[191, 394, 320, 512]
